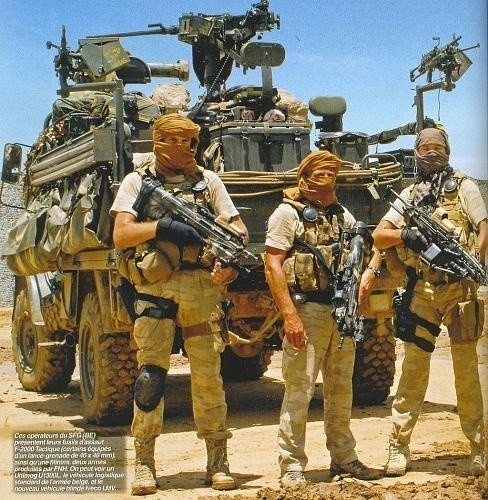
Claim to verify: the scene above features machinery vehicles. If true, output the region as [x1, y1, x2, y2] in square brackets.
[1, 10, 476, 422]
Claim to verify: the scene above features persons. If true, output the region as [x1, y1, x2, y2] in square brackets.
[263, 150, 382, 490]
[373, 127, 487, 476]
[112, 113, 250, 498]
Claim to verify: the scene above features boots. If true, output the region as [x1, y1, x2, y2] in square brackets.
[131, 438, 157, 494]
[206, 438, 235, 490]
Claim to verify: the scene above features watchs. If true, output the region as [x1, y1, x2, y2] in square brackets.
[362, 263, 383, 281]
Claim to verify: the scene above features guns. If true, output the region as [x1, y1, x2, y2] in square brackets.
[408, 33, 483, 88]
[333, 221, 375, 351]
[132, 172, 260, 278]
[380, 180, 488, 288]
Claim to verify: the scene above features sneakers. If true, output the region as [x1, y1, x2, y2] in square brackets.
[330, 460, 386, 480]
[384, 445, 411, 476]
[468, 448, 488, 476]
[281, 471, 306, 487]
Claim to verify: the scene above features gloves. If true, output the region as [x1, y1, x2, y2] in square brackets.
[401, 228, 430, 252]
[156, 217, 203, 248]
